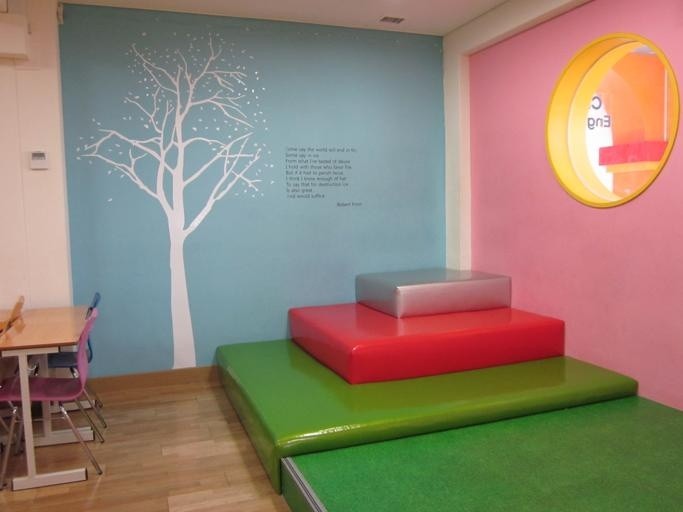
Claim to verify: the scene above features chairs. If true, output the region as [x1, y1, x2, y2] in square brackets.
[0, 293, 108, 492]
